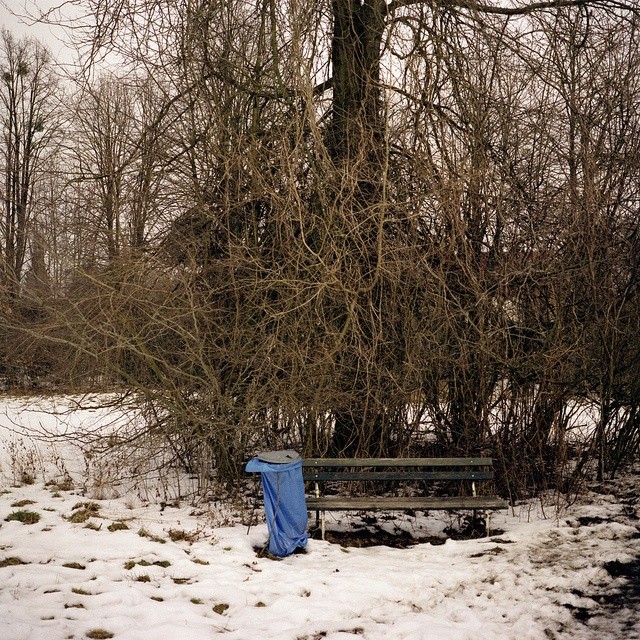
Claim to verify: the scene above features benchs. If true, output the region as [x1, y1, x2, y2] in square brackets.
[301, 458, 509, 541]
